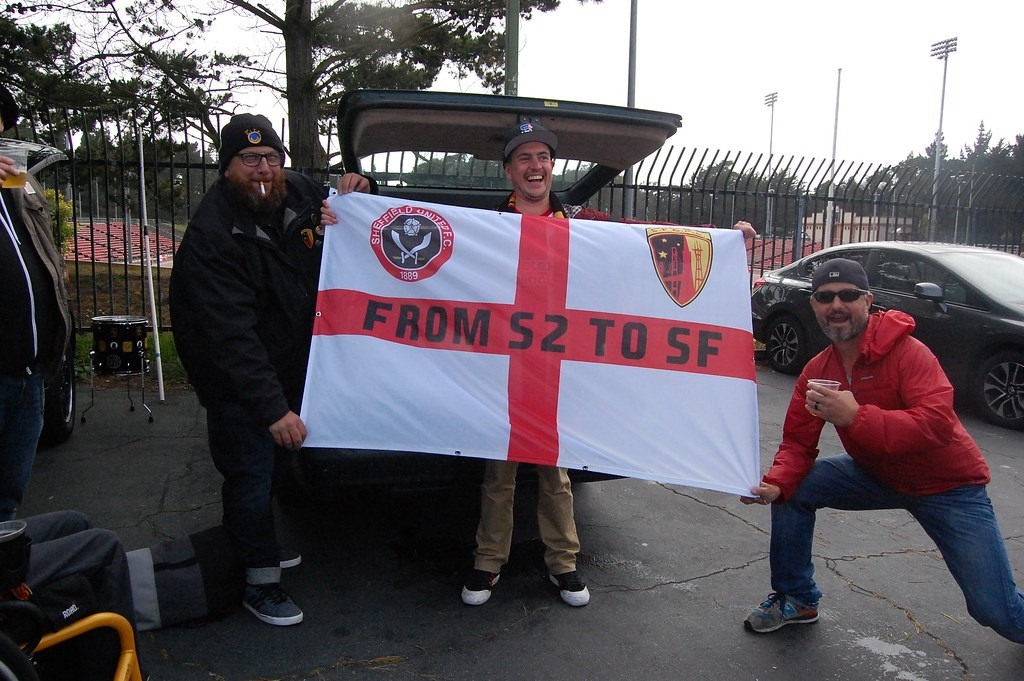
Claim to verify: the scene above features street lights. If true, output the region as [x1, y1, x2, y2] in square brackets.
[763, 92, 780, 221]
[928, 36, 961, 242]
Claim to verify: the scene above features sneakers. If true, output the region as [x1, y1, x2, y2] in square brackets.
[241, 584, 304, 625]
[550, 569, 589, 606]
[743, 592, 819, 633]
[276, 547, 301, 568]
[462, 569, 501, 605]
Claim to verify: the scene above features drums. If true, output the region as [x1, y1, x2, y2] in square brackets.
[91, 314, 151, 378]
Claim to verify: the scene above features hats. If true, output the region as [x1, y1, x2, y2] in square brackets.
[502, 121, 558, 160]
[218, 113, 285, 174]
[810, 258, 870, 295]
[0, 84, 19, 132]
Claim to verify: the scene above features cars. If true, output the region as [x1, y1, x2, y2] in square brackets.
[748, 241, 1024, 433]
[332, 84, 684, 473]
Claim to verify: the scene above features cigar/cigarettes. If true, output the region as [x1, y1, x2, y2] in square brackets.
[260, 182, 265, 194]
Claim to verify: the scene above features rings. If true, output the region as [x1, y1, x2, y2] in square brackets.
[814, 402, 819, 410]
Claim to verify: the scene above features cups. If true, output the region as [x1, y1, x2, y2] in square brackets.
[807, 379, 842, 417]
[0, 145, 30, 189]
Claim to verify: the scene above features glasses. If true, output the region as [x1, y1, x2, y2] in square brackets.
[811, 289, 869, 304]
[234, 152, 285, 167]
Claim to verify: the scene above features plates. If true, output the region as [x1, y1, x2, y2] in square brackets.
[0, 521, 28, 544]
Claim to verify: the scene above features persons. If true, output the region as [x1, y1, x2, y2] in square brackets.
[1, 81, 70, 522]
[741, 258, 1024, 645]
[168, 113, 379, 626]
[336, 124, 757, 607]
[1, 510, 152, 681]
